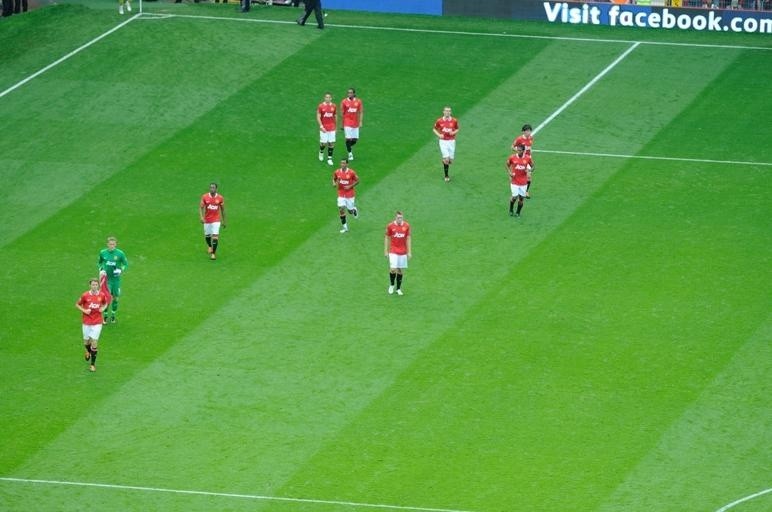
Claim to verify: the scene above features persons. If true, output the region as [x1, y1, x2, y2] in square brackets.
[97, 237, 128, 327]
[119, 0, 133, 14]
[329, 157, 361, 237]
[431, 103, 462, 182]
[297, 1, 328, 29]
[508, 143, 535, 212]
[382, 209, 414, 296]
[312, 93, 339, 163]
[79, 277, 108, 373]
[15, 0, 29, 14]
[338, 88, 363, 158]
[511, 124, 535, 200]
[236, 1, 254, 11]
[3, 1, 13, 18]
[197, 183, 230, 260]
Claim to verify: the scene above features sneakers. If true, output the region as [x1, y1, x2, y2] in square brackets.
[86, 315, 115, 372]
[396, 289, 403, 295]
[208, 245, 212, 253]
[353, 207, 358, 219]
[389, 285, 395, 294]
[211, 254, 216, 260]
[340, 224, 348, 233]
[319, 152, 353, 165]
